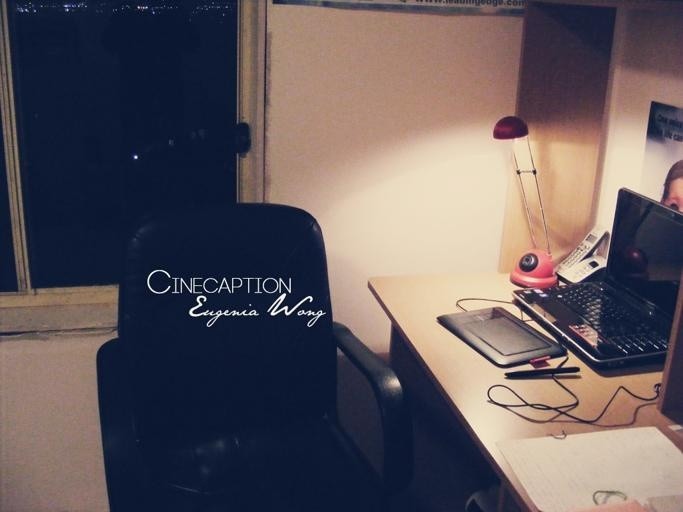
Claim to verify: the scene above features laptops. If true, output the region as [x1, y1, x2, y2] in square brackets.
[511, 186, 683, 371]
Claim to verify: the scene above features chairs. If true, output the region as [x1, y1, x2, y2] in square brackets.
[95, 199, 412, 512]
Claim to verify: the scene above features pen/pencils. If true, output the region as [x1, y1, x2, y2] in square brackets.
[504, 367, 580, 379]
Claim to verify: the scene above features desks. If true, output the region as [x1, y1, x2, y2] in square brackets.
[366, 270, 683, 512]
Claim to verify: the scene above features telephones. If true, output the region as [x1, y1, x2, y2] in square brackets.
[555, 226, 608, 283]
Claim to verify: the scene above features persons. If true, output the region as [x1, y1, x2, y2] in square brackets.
[660, 160, 683, 215]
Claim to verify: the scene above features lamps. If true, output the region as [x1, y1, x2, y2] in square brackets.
[491, 116, 559, 290]
